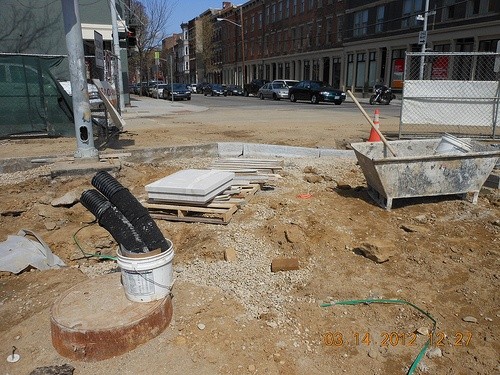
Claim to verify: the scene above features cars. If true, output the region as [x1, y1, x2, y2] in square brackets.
[129, 81, 191, 100]
[0, 63, 68, 124]
[186, 82, 243, 96]
[288, 80, 346, 105]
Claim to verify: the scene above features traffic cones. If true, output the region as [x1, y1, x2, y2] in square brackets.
[366, 107, 382, 142]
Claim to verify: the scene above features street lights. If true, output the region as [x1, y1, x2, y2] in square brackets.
[217, 6, 246, 84]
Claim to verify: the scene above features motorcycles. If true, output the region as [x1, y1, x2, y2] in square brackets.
[370, 84, 396, 105]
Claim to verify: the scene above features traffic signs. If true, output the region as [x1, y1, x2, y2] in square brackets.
[419, 31, 426, 44]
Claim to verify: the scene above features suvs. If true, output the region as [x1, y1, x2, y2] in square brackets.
[244, 79, 300, 101]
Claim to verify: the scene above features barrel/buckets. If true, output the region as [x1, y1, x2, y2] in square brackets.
[115, 238, 175, 302]
